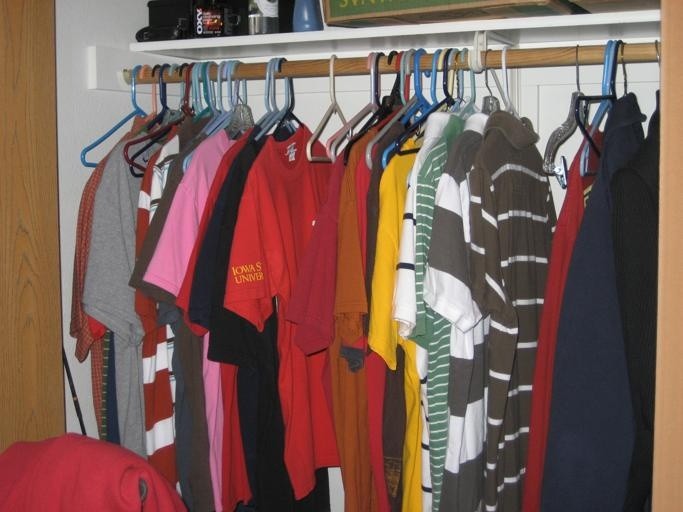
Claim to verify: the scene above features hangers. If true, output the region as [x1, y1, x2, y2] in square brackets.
[77, 42, 642, 181]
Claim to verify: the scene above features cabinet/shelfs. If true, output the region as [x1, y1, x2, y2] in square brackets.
[108, 0, 683, 512]
